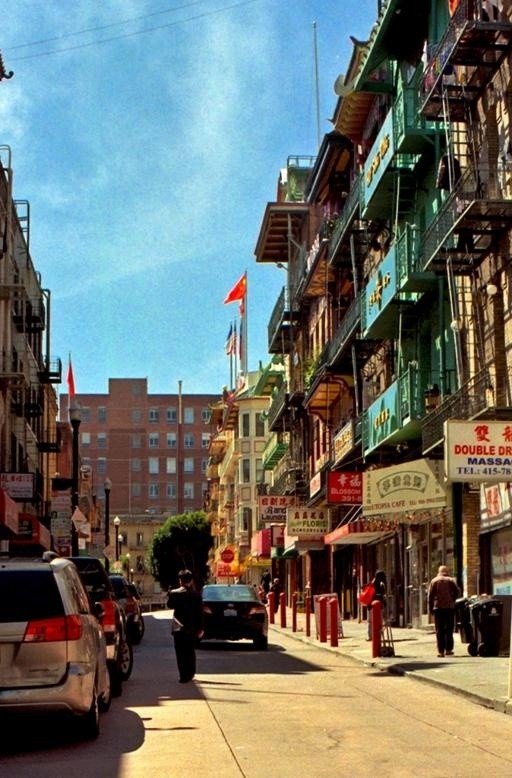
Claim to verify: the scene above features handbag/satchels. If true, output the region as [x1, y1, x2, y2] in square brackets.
[358, 584, 375, 606]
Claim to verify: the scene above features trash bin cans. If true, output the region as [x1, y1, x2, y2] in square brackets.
[454, 594, 511, 657]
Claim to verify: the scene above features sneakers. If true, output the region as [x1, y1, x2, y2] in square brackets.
[446, 651, 454, 655]
[437, 654, 444, 657]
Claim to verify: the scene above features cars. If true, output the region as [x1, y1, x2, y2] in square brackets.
[197, 584, 269, 651]
[1, 550, 146, 742]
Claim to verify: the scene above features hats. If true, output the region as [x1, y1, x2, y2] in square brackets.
[439, 566, 448, 574]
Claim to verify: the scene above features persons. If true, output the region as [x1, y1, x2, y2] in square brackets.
[166, 569, 204, 683]
[365, 569, 387, 640]
[270, 578, 282, 612]
[427, 565, 460, 657]
[253, 568, 272, 600]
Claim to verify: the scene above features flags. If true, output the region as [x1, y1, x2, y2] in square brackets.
[67, 360, 75, 399]
[224, 274, 247, 360]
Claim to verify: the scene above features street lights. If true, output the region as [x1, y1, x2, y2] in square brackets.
[117, 534, 124, 556]
[114, 516, 121, 561]
[126, 552, 131, 582]
[103, 477, 112, 575]
[68, 400, 82, 558]
[130, 568, 134, 583]
